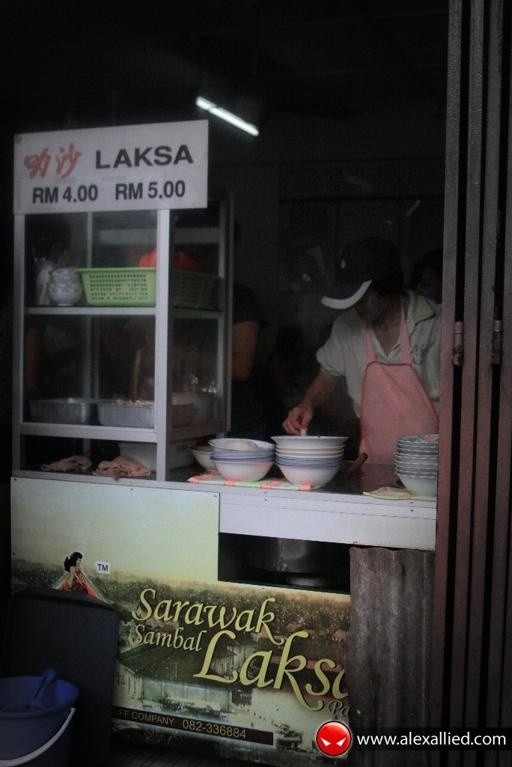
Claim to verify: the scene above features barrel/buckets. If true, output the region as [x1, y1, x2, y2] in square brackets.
[1, 675, 77, 765]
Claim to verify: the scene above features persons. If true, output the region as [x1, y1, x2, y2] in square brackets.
[281, 236, 441, 464]
[64, 552, 88, 595]
[103, 208, 271, 441]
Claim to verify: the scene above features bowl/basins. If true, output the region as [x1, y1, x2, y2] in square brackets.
[393, 433, 438, 498]
[191, 435, 349, 488]
[49, 267, 83, 307]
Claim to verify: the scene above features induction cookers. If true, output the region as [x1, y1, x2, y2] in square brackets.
[0, 666, 59, 714]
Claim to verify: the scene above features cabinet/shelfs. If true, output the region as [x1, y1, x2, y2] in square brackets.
[14, 171, 238, 486]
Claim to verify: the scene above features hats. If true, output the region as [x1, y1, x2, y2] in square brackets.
[317, 236, 400, 311]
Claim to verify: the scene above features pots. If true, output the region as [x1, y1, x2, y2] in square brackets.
[244, 535, 346, 576]
